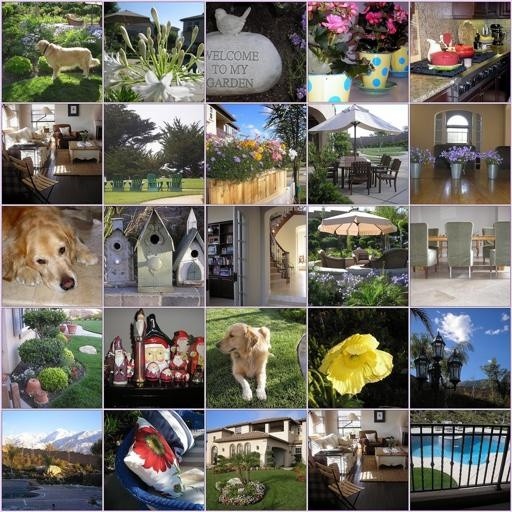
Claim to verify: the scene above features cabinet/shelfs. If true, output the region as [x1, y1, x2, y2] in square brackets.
[207, 220, 237, 299]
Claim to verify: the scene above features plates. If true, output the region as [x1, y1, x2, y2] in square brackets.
[353, 79, 399, 94]
[389, 67, 408, 78]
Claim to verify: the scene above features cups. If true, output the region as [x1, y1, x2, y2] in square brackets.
[463, 59, 473, 68]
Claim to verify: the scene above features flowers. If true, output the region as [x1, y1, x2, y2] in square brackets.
[205, 130, 298, 184]
[410, 143, 504, 179]
[308, 1, 409, 103]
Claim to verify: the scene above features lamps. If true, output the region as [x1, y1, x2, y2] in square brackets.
[2, 104, 16, 121]
[414, 332, 463, 391]
[309, 411, 321, 425]
[36, 107, 55, 129]
[342, 413, 358, 435]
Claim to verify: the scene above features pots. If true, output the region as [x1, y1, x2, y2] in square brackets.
[455, 43, 475, 57]
[430, 49, 459, 65]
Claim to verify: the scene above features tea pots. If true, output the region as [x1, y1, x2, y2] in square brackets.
[425, 38, 443, 62]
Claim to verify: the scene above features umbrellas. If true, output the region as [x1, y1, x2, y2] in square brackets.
[317, 211, 397, 250]
[309, 103, 403, 162]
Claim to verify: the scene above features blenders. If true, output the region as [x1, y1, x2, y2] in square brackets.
[479, 24, 495, 50]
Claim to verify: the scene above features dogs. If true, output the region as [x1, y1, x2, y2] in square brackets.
[215, 323, 274, 402]
[2, 206, 99, 293]
[34, 39, 101, 81]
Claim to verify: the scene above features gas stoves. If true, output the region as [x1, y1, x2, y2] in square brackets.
[409, 51, 504, 100]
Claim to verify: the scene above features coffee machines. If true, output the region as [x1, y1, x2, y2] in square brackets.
[489, 24, 505, 46]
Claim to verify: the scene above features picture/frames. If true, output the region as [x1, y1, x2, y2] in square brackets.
[68, 104, 79, 116]
[374, 411, 385, 422]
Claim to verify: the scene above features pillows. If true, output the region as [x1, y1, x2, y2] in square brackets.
[145, 410, 196, 462]
[123, 417, 186, 498]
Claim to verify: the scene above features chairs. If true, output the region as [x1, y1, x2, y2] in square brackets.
[364, 248, 408, 269]
[318, 250, 346, 268]
[312, 154, 402, 196]
[411, 221, 511, 279]
[2, 124, 102, 204]
[115, 410, 206, 511]
[352, 247, 370, 264]
[308, 430, 408, 510]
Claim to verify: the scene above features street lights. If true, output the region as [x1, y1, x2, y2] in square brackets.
[414, 331, 463, 408]
